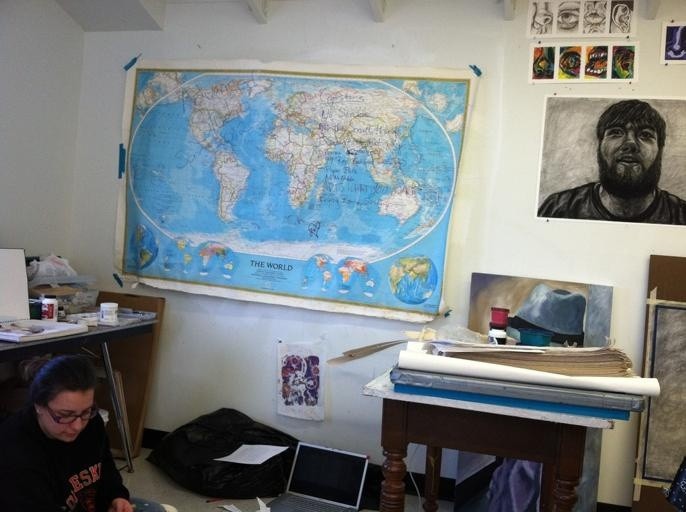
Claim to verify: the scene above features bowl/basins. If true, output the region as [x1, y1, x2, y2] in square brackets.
[517, 328, 554, 346]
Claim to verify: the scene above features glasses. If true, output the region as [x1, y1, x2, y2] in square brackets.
[42, 396, 100, 426]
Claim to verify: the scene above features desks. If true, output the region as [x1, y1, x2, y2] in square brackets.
[0, 313, 161, 474]
[361, 366, 615, 512]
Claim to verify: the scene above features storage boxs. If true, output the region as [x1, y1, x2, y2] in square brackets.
[87, 288, 166, 459]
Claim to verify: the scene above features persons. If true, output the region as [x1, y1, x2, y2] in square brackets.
[540, 99, 686, 228]
[0, 350, 166, 512]
[527, 0, 634, 82]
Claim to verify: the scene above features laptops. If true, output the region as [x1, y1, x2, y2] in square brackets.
[266, 442, 369, 512]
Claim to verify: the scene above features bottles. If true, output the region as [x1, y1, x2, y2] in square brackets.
[41, 294, 59, 322]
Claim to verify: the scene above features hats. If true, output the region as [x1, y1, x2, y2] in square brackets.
[491, 285, 587, 348]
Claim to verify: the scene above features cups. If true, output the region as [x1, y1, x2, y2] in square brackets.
[99, 301, 119, 324]
[488, 306, 510, 344]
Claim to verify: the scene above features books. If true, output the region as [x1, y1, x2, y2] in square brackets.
[389, 365, 646, 421]
[0, 318, 88, 344]
